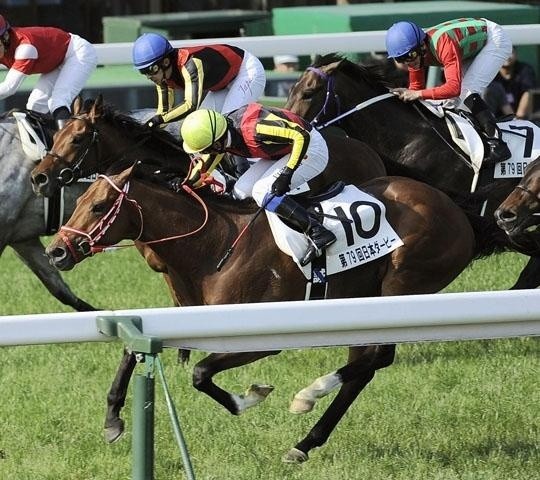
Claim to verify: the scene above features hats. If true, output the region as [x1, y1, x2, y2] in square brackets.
[272, 54, 301, 65]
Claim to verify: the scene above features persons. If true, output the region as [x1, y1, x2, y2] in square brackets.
[497, 46, 537, 121]
[268, 54, 302, 98]
[478, 81, 518, 126]
[132, 32, 266, 178]
[386, 18, 512, 167]
[1, 14, 97, 130]
[180, 102, 336, 266]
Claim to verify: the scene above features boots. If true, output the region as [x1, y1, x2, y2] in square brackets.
[464, 92, 511, 167]
[232, 154, 250, 177]
[273, 196, 338, 268]
[56, 119, 74, 131]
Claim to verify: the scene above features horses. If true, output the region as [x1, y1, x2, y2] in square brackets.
[280, 50, 540, 289]
[28, 90, 414, 364]
[43, 160, 492, 465]
[0, 105, 184, 312]
[494, 155, 540, 238]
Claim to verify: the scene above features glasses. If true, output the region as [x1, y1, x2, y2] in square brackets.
[138, 64, 162, 75]
[394, 51, 419, 64]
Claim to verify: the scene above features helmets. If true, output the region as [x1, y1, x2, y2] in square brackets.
[0, 14, 10, 35]
[131, 32, 175, 70]
[180, 109, 229, 154]
[384, 20, 428, 61]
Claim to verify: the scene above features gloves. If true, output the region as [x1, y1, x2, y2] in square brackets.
[142, 115, 163, 128]
[271, 167, 294, 198]
